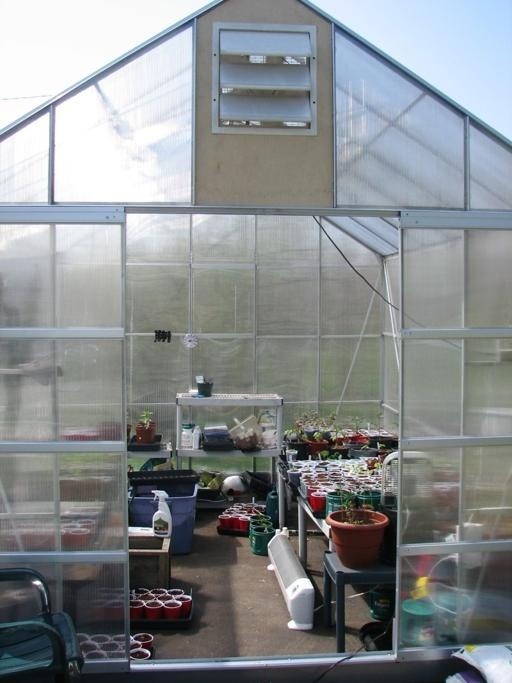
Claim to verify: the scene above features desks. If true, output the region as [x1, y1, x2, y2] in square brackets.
[323, 552, 395, 651]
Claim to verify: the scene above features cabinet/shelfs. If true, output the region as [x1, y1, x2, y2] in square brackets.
[176, 388, 284, 531]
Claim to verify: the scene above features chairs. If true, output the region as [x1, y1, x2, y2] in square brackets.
[0, 567, 85, 683]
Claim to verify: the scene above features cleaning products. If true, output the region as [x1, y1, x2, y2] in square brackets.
[151, 489, 173, 538]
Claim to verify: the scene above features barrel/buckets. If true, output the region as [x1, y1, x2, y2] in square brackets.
[192, 426, 202, 450]
[181, 428, 193, 448]
[432, 590, 473, 638]
[401, 597, 436, 645]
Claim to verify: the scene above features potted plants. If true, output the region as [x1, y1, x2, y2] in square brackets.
[283, 408, 484, 571]
[197, 375, 213, 396]
[135, 409, 157, 445]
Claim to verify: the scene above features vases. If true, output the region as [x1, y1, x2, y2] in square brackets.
[86, 586, 195, 629]
[77, 632, 154, 664]
[215, 501, 276, 556]
[1, 496, 106, 550]
